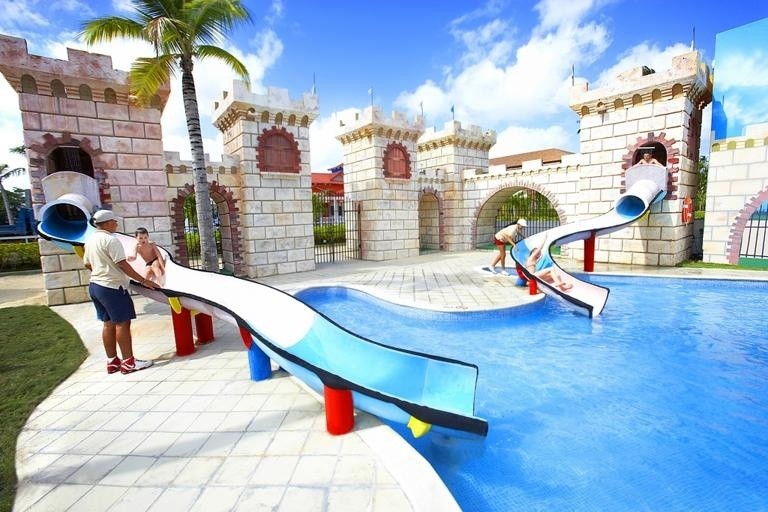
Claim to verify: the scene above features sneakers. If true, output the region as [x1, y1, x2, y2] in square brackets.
[502, 271, 510, 276]
[488, 266, 497, 274]
[120, 356, 154, 374]
[106, 357, 121, 374]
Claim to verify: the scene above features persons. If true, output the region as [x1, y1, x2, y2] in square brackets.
[488, 218, 528, 276]
[82, 209, 162, 375]
[524, 231, 574, 291]
[632, 151, 664, 167]
[125, 227, 166, 286]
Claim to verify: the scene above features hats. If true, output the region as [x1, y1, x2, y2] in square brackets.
[93, 209, 118, 223]
[517, 219, 527, 226]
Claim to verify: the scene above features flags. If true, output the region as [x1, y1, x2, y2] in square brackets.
[366, 88, 373, 96]
[449, 105, 454, 113]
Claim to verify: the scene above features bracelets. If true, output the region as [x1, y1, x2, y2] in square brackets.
[139, 278, 147, 286]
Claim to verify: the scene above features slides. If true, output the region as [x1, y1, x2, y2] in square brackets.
[510, 180, 659, 319]
[34, 194, 488, 439]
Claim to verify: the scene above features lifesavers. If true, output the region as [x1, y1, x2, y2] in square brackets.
[682, 198, 693, 223]
